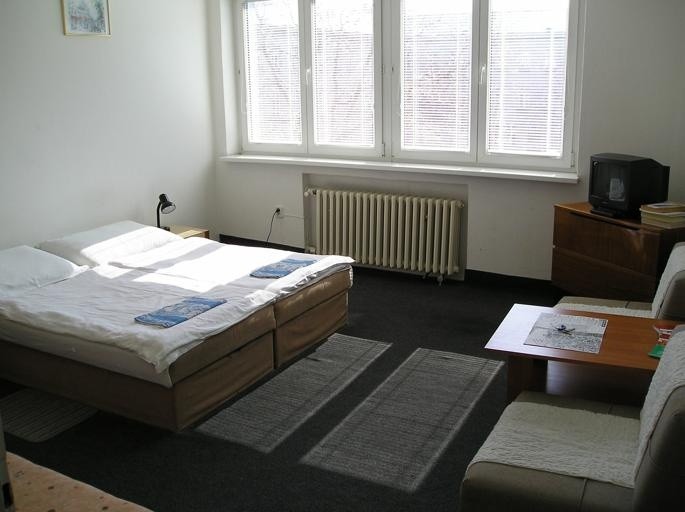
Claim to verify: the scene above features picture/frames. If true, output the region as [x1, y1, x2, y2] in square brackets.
[59, 0, 112, 39]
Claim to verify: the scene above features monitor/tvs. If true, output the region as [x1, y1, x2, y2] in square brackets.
[589, 152, 669, 220]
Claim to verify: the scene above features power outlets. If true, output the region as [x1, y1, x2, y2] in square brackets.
[274, 205, 283, 219]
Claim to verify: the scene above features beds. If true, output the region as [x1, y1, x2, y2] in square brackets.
[1, 219, 352, 433]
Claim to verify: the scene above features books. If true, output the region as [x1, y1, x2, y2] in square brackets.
[641, 200, 685, 230]
[649, 325, 677, 360]
[524, 312, 610, 354]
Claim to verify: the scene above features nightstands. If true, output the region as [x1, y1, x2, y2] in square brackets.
[167, 225, 209, 239]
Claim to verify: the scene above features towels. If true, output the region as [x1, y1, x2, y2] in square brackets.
[133, 295, 226, 327]
[250, 253, 325, 279]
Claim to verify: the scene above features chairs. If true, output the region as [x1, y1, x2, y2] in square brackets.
[461, 327, 683, 510]
[555, 243, 684, 309]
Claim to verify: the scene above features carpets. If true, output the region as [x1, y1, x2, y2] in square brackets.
[308, 344, 517, 486]
[212, 333, 399, 454]
[0, 387, 104, 441]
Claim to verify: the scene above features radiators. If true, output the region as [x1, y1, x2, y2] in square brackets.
[305, 186, 463, 282]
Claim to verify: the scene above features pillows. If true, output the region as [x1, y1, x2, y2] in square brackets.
[41, 220, 179, 267]
[0, 241, 90, 298]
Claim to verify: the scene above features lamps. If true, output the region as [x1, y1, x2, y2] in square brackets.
[155, 190, 174, 232]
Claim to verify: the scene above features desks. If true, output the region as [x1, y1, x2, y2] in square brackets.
[484, 303, 677, 400]
[553, 202, 685, 297]
